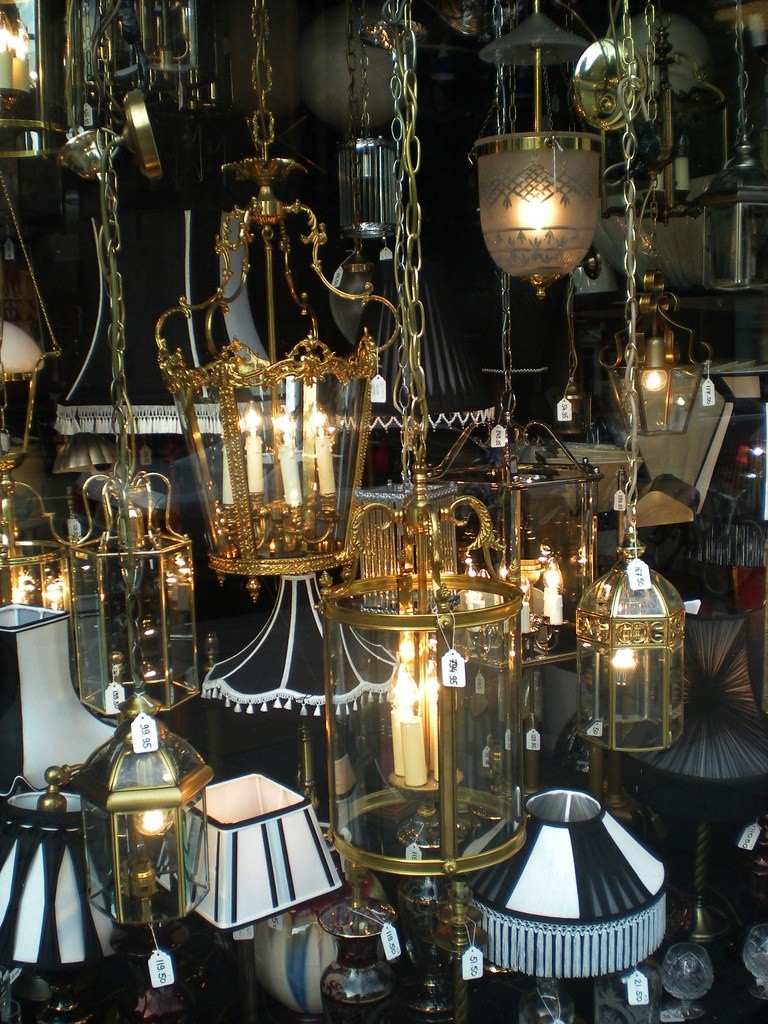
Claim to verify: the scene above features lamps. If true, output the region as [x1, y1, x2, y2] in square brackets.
[0, 0, 768, 1024]
